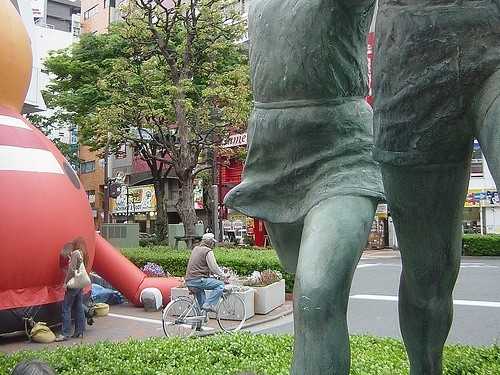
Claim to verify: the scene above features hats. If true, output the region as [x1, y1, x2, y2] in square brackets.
[202, 233, 217, 242]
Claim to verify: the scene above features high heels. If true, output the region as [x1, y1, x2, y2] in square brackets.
[72, 333, 83, 338]
[56, 335, 69, 341]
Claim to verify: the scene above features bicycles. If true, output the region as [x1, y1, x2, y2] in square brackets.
[161, 277, 248, 339]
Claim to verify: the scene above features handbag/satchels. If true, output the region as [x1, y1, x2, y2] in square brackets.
[66, 250, 91, 289]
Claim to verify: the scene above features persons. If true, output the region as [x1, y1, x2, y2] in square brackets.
[186, 233, 228, 332]
[52, 237, 90, 341]
[221, 0, 499, 374]
[13, 359, 59, 375]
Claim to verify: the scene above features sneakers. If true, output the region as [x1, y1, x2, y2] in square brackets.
[192, 327, 203, 331]
[202, 303, 218, 313]
